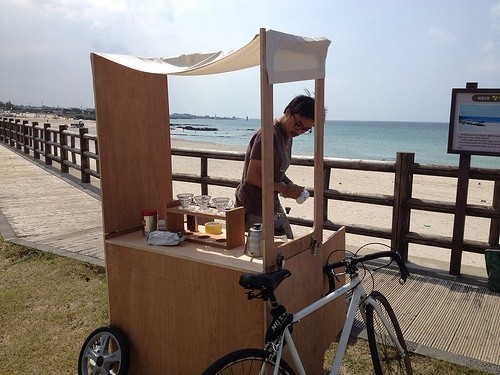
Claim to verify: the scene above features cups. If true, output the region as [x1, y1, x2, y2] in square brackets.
[194, 195, 214, 210]
[296, 189, 310, 204]
[212, 198, 234, 215]
[177, 193, 193, 209]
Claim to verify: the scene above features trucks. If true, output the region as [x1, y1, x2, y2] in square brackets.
[70, 121, 84, 128]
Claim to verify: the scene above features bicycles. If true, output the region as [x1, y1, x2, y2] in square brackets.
[199, 242, 414, 375]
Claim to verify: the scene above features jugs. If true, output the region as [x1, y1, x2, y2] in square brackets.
[243, 223, 263, 257]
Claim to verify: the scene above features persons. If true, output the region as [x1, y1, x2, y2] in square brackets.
[234, 88, 328, 240]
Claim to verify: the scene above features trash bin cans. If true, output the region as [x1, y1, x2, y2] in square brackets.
[484, 249, 500, 291]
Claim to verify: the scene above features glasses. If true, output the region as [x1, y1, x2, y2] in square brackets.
[291, 113, 312, 135]
